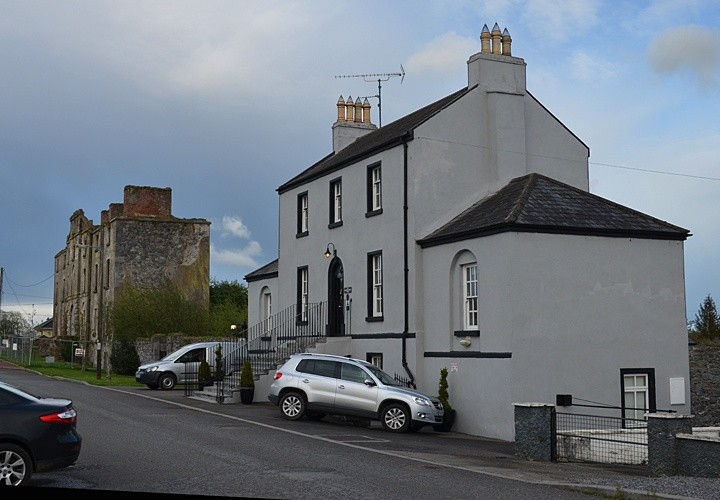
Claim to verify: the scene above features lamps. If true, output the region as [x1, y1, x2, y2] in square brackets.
[324, 243, 337, 258]
[459, 340, 471, 346]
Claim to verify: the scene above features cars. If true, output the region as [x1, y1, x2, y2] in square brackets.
[0, 382, 82, 486]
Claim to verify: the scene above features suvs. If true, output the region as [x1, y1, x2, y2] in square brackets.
[267, 353, 444, 434]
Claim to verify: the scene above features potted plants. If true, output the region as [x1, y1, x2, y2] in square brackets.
[198, 361, 214, 391]
[240, 360, 255, 404]
[429, 367, 456, 432]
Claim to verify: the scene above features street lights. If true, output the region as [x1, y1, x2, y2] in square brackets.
[76, 244, 104, 380]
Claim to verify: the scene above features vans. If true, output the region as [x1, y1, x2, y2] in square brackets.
[134, 341, 248, 390]
